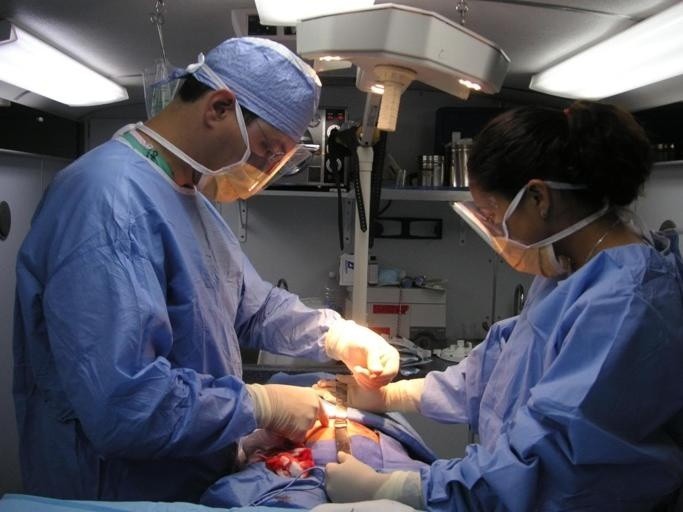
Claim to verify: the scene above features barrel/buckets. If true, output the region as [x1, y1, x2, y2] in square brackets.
[447, 142, 474, 188]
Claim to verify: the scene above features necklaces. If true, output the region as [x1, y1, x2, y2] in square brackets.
[582, 219, 621, 264]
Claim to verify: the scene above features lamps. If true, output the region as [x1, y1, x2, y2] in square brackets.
[524, 0, 683, 102]
[292, 0, 512, 326]
[0, 22, 130, 111]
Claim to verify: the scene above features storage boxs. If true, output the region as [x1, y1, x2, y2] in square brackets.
[343, 282, 449, 351]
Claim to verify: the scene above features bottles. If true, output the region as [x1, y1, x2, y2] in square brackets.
[323, 271, 341, 308]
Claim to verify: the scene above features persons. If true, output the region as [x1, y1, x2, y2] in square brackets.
[9, 35, 401, 510]
[198, 368, 434, 511]
[310, 101, 683, 509]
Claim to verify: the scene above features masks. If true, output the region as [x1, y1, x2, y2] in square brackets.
[195, 160, 272, 204]
[491, 236, 573, 278]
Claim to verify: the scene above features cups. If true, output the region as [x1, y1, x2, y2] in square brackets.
[449, 139, 473, 190]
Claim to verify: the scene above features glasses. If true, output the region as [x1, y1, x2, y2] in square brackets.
[250, 114, 297, 164]
